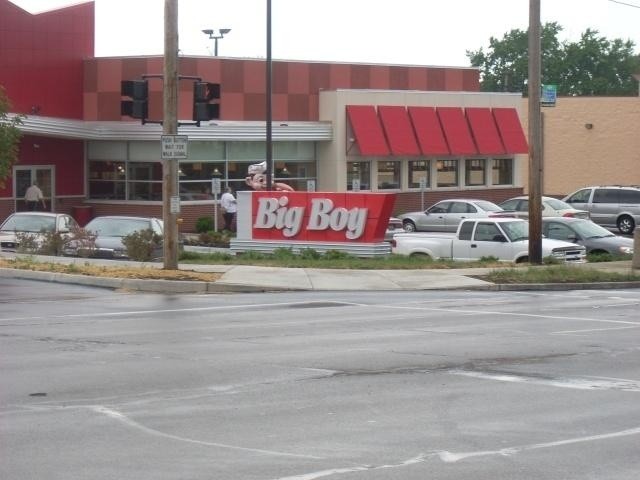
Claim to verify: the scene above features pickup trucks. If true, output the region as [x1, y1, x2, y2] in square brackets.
[391, 217, 587, 264]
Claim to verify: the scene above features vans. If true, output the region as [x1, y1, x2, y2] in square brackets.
[560, 184, 640, 235]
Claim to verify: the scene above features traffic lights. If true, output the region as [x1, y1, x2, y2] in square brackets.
[119, 78, 149, 119]
[192, 81, 220, 123]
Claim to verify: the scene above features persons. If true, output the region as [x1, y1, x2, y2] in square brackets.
[23, 180, 48, 211]
[220, 187, 236, 231]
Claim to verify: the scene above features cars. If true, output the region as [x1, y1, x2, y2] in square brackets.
[59, 215, 164, 262]
[0, 211, 81, 256]
[497, 195, 591, 220]
[395, 199, 529, 232]
[541, 217, 634, 256]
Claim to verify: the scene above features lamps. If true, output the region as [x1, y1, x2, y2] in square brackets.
[179, 170, 187, 177]
[118, 163, 123, 170]
[280, 168, 289, 175]
[118, 169, 126, 175]
[211, 168, 223, 176]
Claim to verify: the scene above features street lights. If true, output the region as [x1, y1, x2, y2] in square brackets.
[210, 168, 224, 234]
[201, 29, 232, 56]
